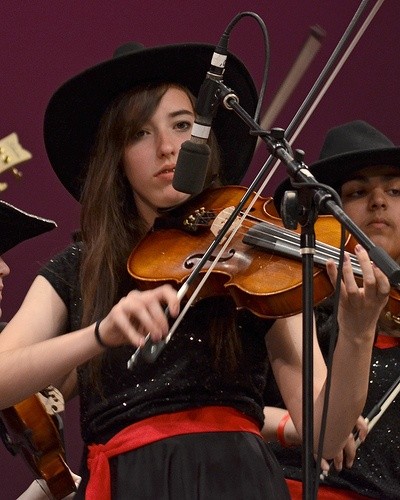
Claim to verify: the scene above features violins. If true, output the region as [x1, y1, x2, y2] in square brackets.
[0, 320, 79, 500]
[127, 185, 400, 324]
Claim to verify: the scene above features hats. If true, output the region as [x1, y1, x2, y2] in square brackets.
[274, 120, 400, 219]
[43, 43, 259, 203]
[0, 200, 58, 257]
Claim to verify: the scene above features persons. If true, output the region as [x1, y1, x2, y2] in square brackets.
[261, 120, 400, 500]
[0, 43, 392, 500]
[0, 199, 81, 500]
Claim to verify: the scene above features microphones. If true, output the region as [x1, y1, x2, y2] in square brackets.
[172, 33, 230, 194]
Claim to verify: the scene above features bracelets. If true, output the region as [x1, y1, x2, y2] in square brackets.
[94, 320, 111, 350]
[278, 413, 293, 450]
